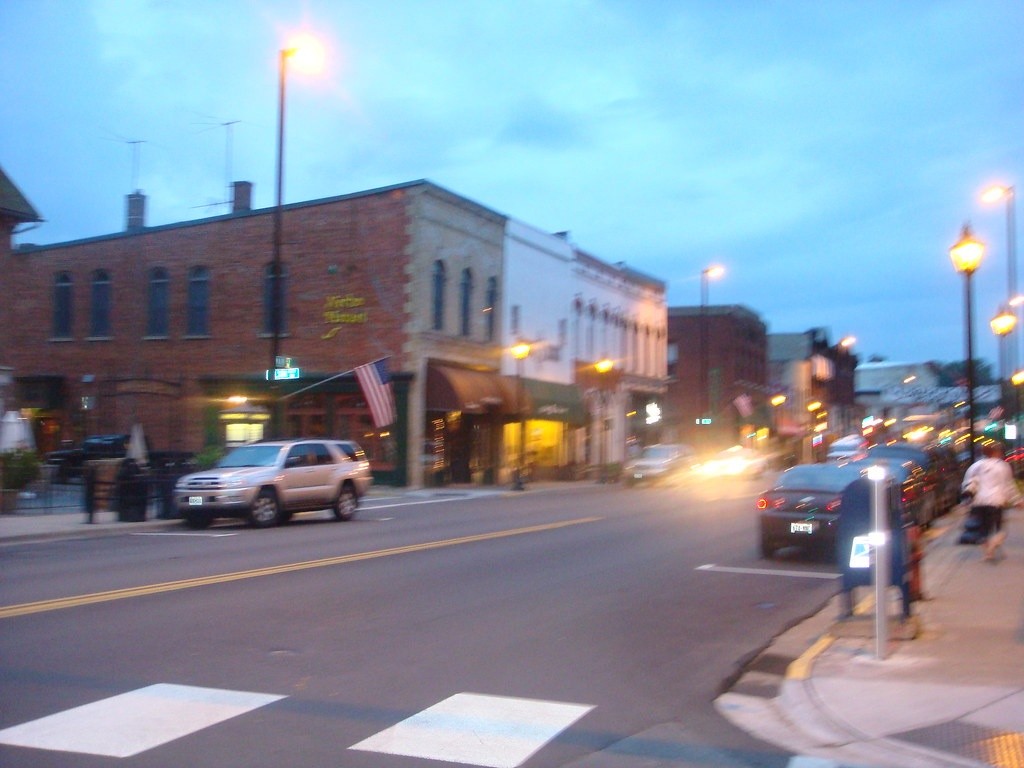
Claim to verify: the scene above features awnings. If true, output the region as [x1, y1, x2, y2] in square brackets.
[425, 357, 531, 414]
[521, 378, 583, 423]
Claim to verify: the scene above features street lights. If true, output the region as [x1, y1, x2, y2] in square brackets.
[697, 262, 727, 458]
[978, 181, 1018, 421]
[595, 355, 615, 483]
[511, 341, 533, 493]
[267, 34, 319, 431]
[949, 224, 988, 465]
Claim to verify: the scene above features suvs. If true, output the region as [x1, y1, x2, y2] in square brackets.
[171, 436, 375, 528]
[621, 445, 696, 487]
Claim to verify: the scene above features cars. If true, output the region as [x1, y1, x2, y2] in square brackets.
[826, 434, 869, 461]
[755, 443, 962, 565]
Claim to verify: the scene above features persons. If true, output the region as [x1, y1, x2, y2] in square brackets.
[957, 445, 1022, 562]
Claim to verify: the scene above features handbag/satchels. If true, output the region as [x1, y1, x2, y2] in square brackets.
[961, 477, 978, 496]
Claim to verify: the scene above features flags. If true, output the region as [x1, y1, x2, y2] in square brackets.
[731, 393, 755, 418]
[355, 354, 398, 429]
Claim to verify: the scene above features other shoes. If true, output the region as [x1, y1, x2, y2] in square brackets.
[980, 555, 995, 562]
